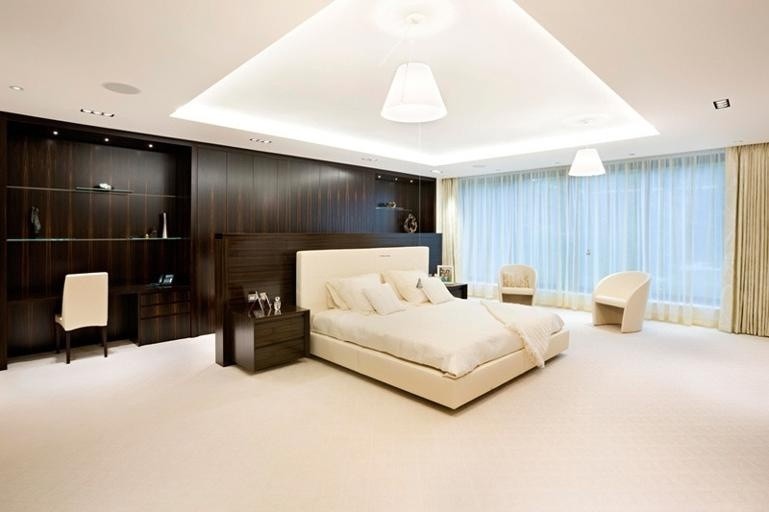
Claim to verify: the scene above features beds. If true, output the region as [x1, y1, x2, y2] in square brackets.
[295, 245, 571, 411]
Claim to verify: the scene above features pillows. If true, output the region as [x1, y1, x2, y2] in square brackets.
[326, 274, 384, 318]
[383, 270, 430, 305]
[364, 283, 407, 318]
[420, 277, 455, 305]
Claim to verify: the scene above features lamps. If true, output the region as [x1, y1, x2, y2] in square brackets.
[379, 60, 449, 126]
[568, 147, 607, 178]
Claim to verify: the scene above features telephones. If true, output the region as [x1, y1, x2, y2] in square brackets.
[144, 273, 174, 287]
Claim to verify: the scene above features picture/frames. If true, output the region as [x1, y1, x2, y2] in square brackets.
[436, 264, 455, 285]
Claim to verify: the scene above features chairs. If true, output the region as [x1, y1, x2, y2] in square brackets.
[591, 270, 653, 335]
[54, 271, 110, 366]
[497, 262, 538, 306]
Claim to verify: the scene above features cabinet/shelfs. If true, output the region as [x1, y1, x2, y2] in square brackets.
[0, 112, 194, 372]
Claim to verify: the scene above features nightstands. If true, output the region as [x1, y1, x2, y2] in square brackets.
[444, 284, 468, 301]
[234, 306, 311, 374]
